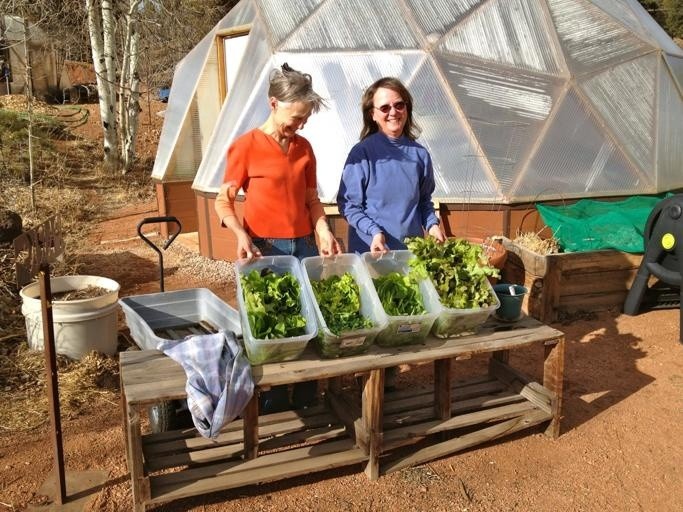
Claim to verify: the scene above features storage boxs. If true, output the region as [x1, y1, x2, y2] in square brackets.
[232, 240, 504, 369]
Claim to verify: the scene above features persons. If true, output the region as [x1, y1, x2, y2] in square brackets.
[336, 75, 449, 389]
[213, 61, 343, 414]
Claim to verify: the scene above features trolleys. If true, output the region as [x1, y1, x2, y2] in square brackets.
[118, 216, 242, 430]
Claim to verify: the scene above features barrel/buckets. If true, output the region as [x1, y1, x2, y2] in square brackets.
[445, 237, 507, 290]
[19, 275, 120, 364]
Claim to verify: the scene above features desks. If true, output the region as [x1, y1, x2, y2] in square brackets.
[118, 310, 570, 508]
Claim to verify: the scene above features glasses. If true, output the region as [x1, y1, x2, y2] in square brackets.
[372, 101, 408, 113]
